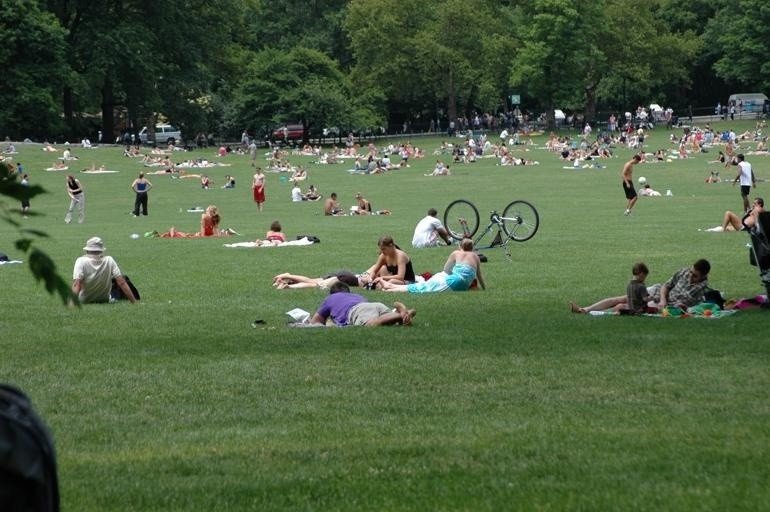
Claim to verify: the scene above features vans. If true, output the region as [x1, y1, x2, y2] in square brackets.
[131, 124, 182, 146]
[262, 125, 312, 141]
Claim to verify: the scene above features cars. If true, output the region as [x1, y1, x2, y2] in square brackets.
[554, 110, 565, 125]
[321, 124, 384, 137]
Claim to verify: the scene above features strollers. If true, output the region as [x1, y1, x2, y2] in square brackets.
[741, 211, 770, 294]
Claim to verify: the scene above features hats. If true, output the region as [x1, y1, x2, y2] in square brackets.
[82, 236, 106, 251]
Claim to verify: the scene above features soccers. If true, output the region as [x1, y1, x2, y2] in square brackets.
[639, 177, 646, 185]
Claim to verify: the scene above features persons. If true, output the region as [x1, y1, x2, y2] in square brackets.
[132, 172, 152, 217]
[267, 124, 425, 216]
[622, 155, 641, 216]
[627, 263, 658, 314]
[369, 236, 415, 289]
[0, 129, 257, 188]
[65, 174, 84, 224]
[380, 239, 486, 292]
[70, 237, 137, 306]
[540, 102, 769, 197]
[570, 259, 714, 314]
[272, 245, 400, 290]
[310, 282, 416, 327]
[411, 209, 453, 246]
[252, 166, 265, 214]
[424, 111, 540, 177]
[20, 174, 31, 219]
[720, 154, 766, 232]
[750, 208, 770, 308]
[171, 204, 233, 237]
[267, 221, 285, 244]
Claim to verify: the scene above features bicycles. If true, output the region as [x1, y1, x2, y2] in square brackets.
[443, 200, 542, 260]
